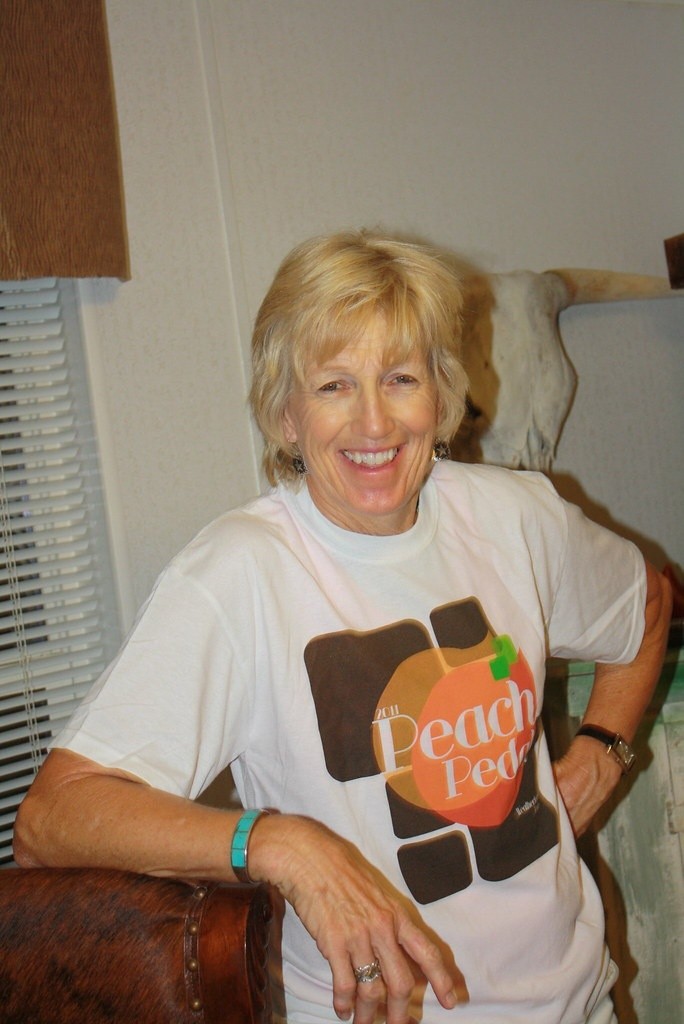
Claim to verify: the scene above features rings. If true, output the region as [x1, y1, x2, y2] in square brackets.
[352, 958, 381, 982]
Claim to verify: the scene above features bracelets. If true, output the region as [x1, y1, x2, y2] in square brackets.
[230, 808, 271, 884]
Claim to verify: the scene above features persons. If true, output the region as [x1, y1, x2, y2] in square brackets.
[11, 237, 678, 1024]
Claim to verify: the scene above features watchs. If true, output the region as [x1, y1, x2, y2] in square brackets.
[576, 723, 638, 775]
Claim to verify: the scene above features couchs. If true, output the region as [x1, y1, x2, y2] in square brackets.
[0, 866, 272, 1024]
